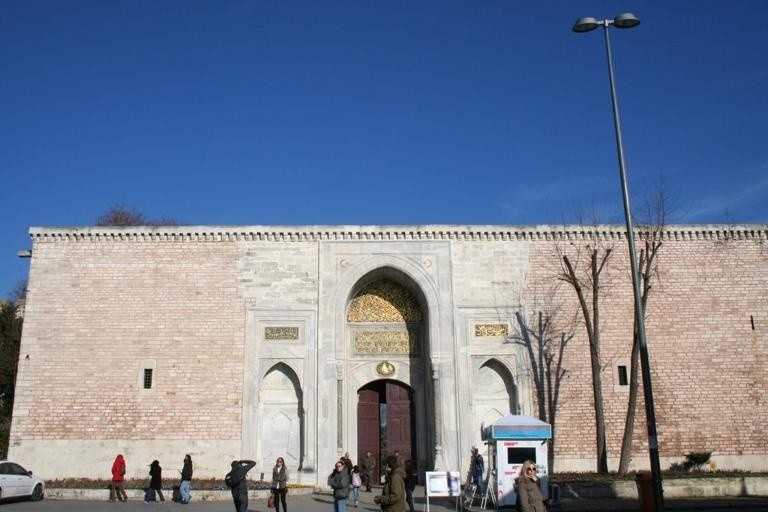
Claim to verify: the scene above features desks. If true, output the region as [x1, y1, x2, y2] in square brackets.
[572, 13, 666, 511]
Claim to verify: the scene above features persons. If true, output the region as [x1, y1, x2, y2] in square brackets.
[177, 454, 192, 504]
[228, 458, 252, 512]
[471, 447, 485, 496]
[142, 459, 166, 505]
[516, 460, 546, 512]
[270, 457, 290, 512]
[328, 450, 420, 511]
[108, 454, 128, 501]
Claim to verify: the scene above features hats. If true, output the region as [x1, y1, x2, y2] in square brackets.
[0, 459, 45, 504]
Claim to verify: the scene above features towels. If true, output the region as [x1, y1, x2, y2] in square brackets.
[636, 472, 655, 509]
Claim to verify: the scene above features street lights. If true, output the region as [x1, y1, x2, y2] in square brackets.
[268, 492, 274, 508]
[119, 464, 126, 476]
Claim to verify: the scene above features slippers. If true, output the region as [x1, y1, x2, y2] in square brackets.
[225, 469, 240, 487]
[351, 471, 362, 488]
